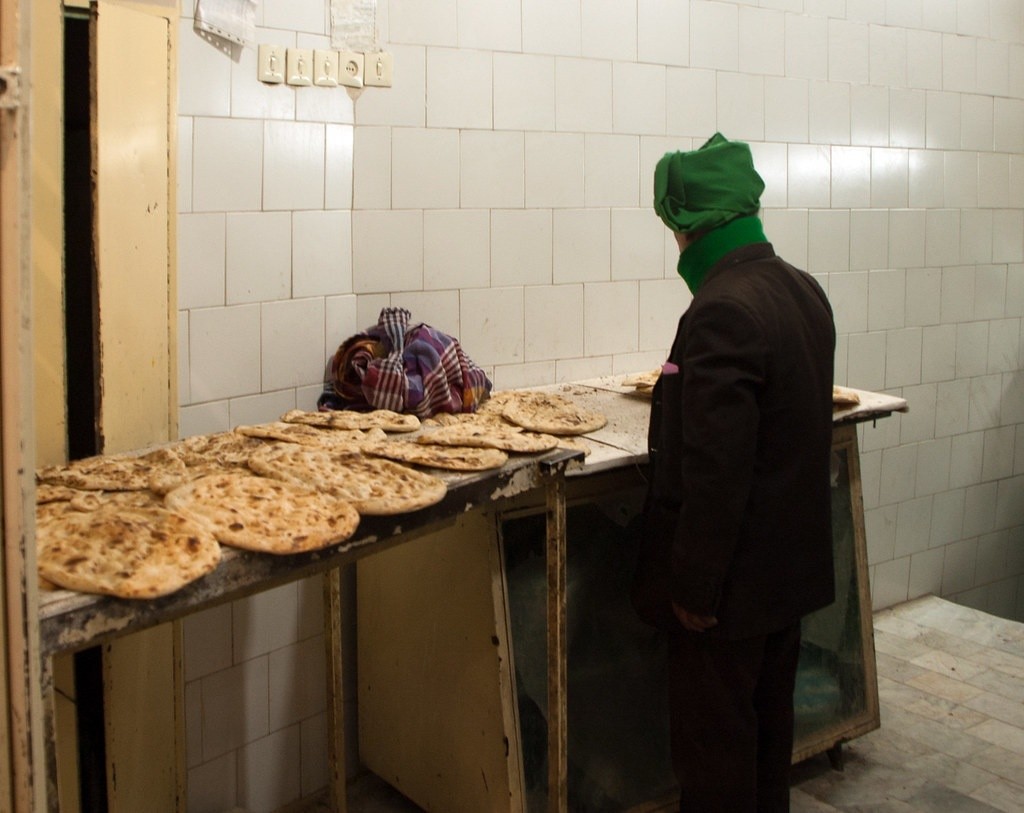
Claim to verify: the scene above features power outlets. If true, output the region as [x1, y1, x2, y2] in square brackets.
[337, 51, 365, 88]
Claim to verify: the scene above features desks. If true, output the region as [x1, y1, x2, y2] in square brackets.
[36, 444, 578, 813]
[354, 373, 908, 813]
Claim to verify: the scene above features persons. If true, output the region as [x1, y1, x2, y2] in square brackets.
[649, 135, 838, 813]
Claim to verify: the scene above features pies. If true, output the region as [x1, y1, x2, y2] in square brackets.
[33, 387, 606, 598]
[621, 366, 662, 394]
[832, 386, 860, 404]
